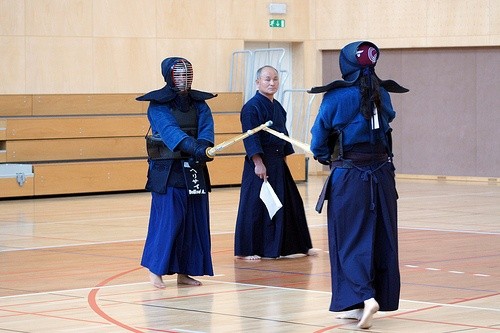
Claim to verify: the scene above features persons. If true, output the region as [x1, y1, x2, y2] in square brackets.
[308, 40, 409, 329]
[234, 65, 313, 260]
[135, 57, 218, 288]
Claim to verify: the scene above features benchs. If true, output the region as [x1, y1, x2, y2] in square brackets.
[0, 92, 309, 198]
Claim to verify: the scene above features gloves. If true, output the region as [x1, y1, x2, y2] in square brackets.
[188, 156, 207, 170]
[195, 144, 215, 163]
